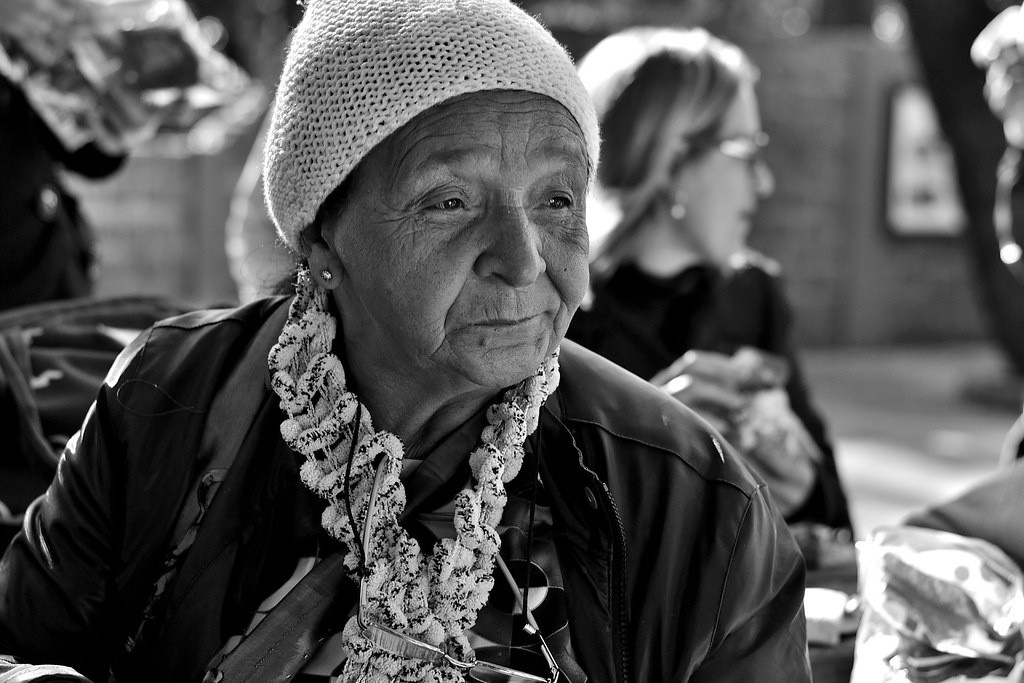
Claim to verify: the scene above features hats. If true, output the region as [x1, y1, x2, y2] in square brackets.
[261, 0, 601, 248]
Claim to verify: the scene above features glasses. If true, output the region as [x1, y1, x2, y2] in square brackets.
[356, 453, 560, 683]
[702, 134, 773, 170]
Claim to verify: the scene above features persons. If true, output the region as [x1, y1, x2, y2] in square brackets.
[0, 0, 1024, 683]
